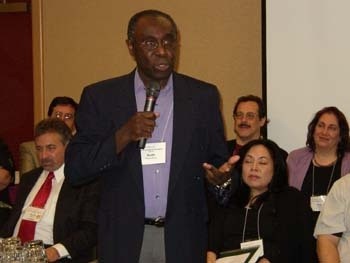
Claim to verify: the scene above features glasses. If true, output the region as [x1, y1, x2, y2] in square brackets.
[52, 112, 74, 119]
[232, 112, 259, 120]
[135, 37, 180, 50]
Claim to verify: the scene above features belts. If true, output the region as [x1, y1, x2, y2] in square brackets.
[144, 216, 165, 227]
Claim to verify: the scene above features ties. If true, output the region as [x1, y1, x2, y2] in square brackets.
[16, 172, 55, 250]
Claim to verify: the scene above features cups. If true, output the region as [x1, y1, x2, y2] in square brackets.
[0, 238, 48, 263]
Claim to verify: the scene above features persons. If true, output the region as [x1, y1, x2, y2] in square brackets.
[218, 96, 289, 162]
[285, 106, 350, 219]
[0, 117, 99, 263]
[64, 9, 240, 263]
[19, 96, 78, 174]
[312, 174, 350, 263]
[0, 128, 16, 229]
[204, 138, 316, 263]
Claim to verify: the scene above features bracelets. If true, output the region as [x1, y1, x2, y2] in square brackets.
[216, 178, 232, 190]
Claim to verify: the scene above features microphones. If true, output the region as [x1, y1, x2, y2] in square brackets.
[136, 79, 161, 150]
